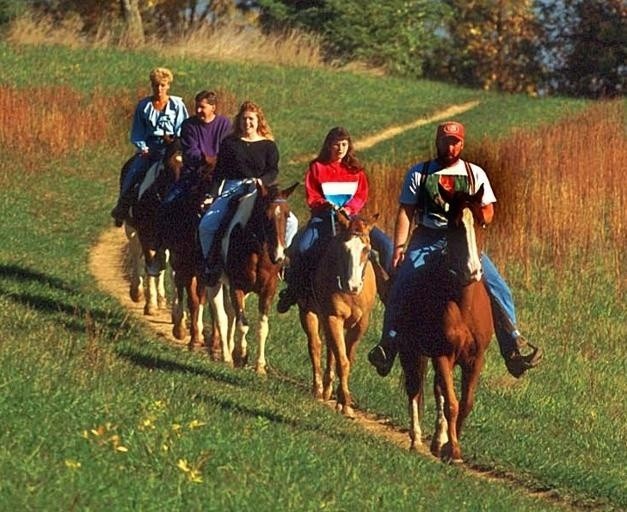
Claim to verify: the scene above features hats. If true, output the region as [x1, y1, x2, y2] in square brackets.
[436, 121, 464, 142]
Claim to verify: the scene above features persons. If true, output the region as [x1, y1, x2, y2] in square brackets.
[155, 90, 233, 226]
[274, 124, 394, 317]
[199, 100, 301, 288]
[110, 65, 191, 227]
[368, 120, 538, 378]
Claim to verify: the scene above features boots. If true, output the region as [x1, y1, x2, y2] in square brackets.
[506, 348, 542, 379]
[368, 341, 397, 376]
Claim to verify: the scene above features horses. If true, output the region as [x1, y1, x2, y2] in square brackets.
[120, 136, 221, 363]
[385, 182, 495, 467]
[286, 210, 379, 421]
[193, 181, 301, 381]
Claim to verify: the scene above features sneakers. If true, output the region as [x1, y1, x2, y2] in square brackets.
[276, 286, 299, 313]
